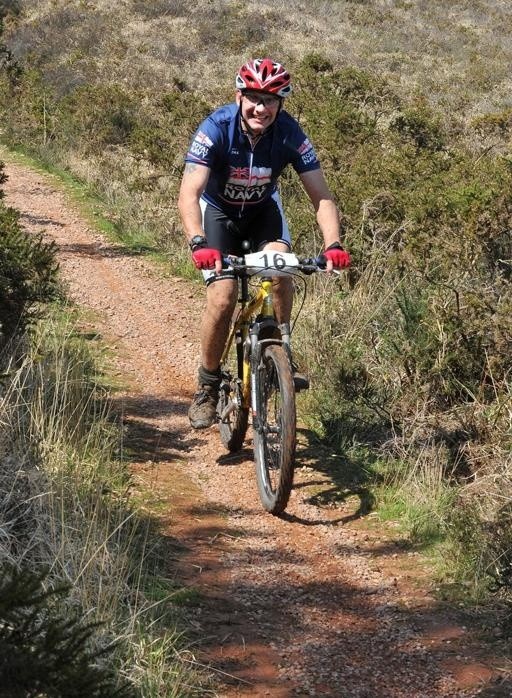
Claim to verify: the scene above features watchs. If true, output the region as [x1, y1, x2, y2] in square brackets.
[188, 234, 208, 249]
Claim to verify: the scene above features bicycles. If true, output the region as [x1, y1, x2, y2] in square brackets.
[202, 248, 342, 518]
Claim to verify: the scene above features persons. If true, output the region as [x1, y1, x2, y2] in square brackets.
[176, 57, 349, 430]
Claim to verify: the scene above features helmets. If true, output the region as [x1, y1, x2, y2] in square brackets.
[235, 58, 293, 97]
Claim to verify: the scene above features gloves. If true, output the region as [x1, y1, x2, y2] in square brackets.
[322, 249, 350, 269]
[191, 244, 221, 271]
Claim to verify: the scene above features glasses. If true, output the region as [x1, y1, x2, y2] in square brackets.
[241, 93, 281, 107]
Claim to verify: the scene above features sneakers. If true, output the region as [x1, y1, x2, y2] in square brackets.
[187, 385, 219, 429]
[274, 357, 308, 391]
